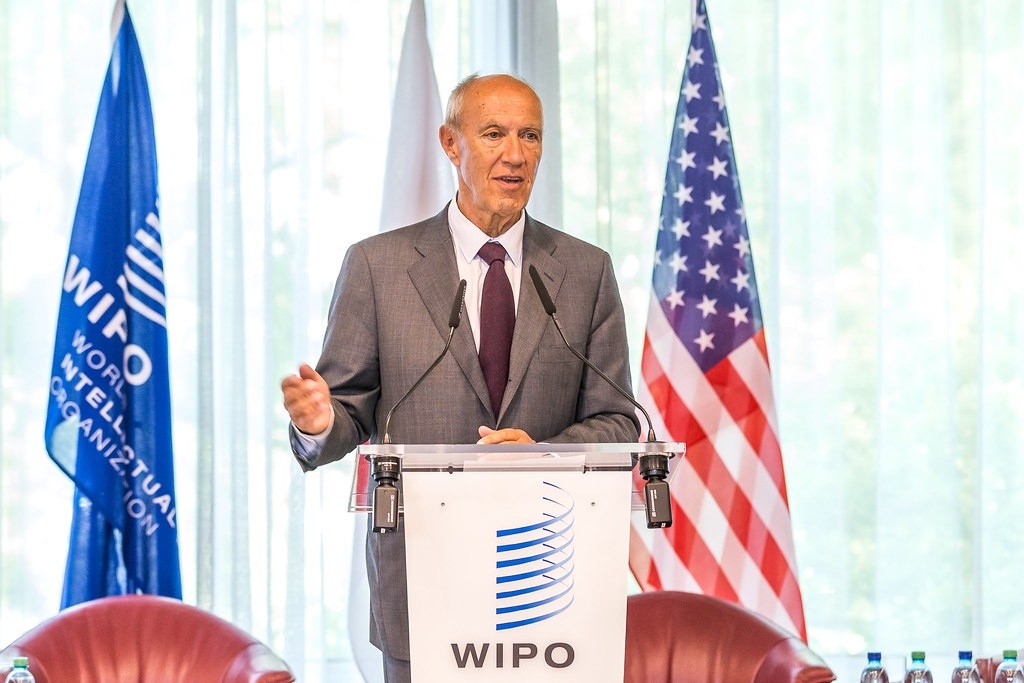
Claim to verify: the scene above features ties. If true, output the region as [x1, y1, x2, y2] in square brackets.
[476, 242, 516, 426]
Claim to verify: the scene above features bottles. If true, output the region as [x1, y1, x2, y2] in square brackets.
[860, 652, 890, 683]
[5, 657, 36, 683]
[904, 651, 934, 683]
[952, 650, 981, 683]
[995, 650, 1024, 683]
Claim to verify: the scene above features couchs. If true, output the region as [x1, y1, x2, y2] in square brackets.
[623, 588, 839, 682]
[0, 591, 296, 683]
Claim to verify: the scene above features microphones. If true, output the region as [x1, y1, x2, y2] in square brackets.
[381, 279, 467, 442]
[528, 264, 658, 442]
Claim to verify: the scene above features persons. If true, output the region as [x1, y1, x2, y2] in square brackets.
[281, 69, 641, 683]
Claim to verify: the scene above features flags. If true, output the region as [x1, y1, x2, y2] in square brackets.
[43, 0, 185, 610]
[336, 0, 456, 683]
[627, 0, 812, 644]
